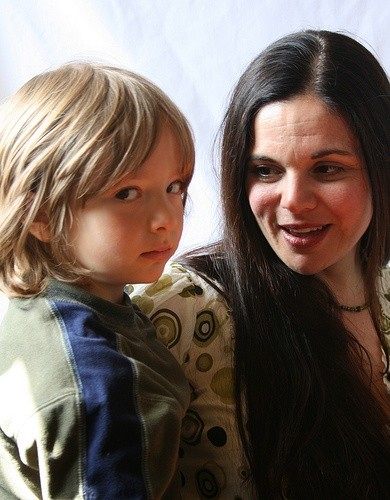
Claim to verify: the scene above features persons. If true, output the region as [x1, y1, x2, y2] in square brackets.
[1, 63, 195, 499]
[125, 30, 390, 500]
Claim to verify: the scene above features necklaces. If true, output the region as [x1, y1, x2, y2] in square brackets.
[335, 298, 372, 313]
